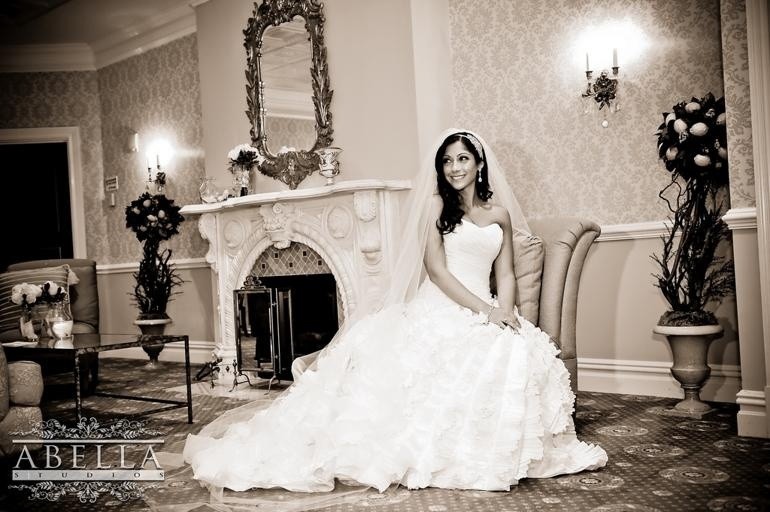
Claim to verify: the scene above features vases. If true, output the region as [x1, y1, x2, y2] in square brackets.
[233, 165, 254, 196]
[652, 324, 727, 421]
[132, 318, 173, 372]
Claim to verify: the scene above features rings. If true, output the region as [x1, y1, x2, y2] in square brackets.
[504, 318, 509, 323]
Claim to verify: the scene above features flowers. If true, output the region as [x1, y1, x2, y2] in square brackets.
[649, 92, 735, 314]
[225, 143, 265, 181]
[123, 190, 193, 315]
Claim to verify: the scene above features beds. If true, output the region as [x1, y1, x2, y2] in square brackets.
[3, 333, 193, 448]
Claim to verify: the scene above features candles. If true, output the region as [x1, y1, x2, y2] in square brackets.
[612, 49, 618, 66]
[156, 154, 159, 166]
[585, 53, 590, 69]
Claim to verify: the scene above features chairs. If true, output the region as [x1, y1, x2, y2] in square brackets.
[2, 258, 102, 390]
[529, 214, 604, 430]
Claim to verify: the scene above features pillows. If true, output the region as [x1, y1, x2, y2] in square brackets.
[513, 233, 544, 329]
[0, 264, 82, 334]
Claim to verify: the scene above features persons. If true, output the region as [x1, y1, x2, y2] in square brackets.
[183, 124, 612, 490]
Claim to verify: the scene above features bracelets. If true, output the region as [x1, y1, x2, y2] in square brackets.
[487, 305, 495, 320]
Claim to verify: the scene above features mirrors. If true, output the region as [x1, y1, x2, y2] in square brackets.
[240, 0, 335, 190]
